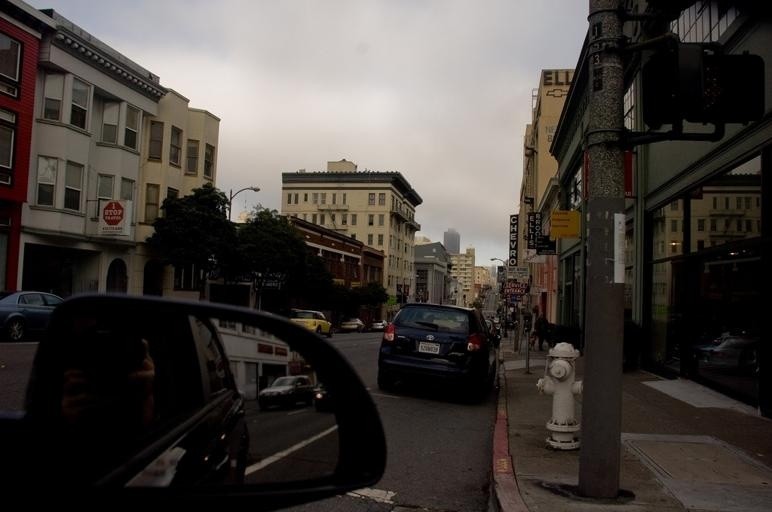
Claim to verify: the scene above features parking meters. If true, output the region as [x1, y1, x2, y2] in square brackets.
[522, 311, 533, 373]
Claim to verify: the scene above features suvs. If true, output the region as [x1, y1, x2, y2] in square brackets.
[257, 374, 315, 411]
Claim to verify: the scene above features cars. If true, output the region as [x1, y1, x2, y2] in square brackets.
[39, 306, 249, 492]
[645, 305, 694, 368]
[377, 302, 500, 404]
[697, 328, 760, 377]
[340, 318, 368, 333]
[288, 309, 333, 338]
[369, 320, 389, 331]
[311, 381, 333, 413]
[484, 313, 502, 348]
[0, 291, 65, 342]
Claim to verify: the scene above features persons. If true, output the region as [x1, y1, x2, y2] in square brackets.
[502, 308, 549, 351]
[61, 340, 228, 497]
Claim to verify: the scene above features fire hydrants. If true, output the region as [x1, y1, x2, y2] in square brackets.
[535, 342, 583, 452]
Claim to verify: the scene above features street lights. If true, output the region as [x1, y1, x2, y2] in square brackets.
[402, 274, 419, 306]
[490, 257, 508, 332]
[222, 186, 260, 304]
